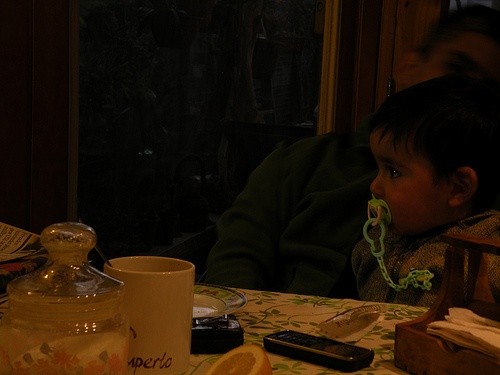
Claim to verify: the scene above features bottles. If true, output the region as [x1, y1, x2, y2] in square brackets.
[0, 222, 130, 375]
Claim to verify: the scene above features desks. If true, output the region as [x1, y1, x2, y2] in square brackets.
[0, 286, 430, 375]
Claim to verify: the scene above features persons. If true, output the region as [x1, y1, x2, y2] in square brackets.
[201, 5, 500, 300]
[349, 70, 500, 314]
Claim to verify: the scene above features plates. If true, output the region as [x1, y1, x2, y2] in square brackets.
[193, 282, 248, 317]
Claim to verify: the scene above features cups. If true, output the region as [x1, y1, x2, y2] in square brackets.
[104, 256, 195, 374]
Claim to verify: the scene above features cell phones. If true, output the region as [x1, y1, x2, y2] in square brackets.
[264, 329, 374, 371]
